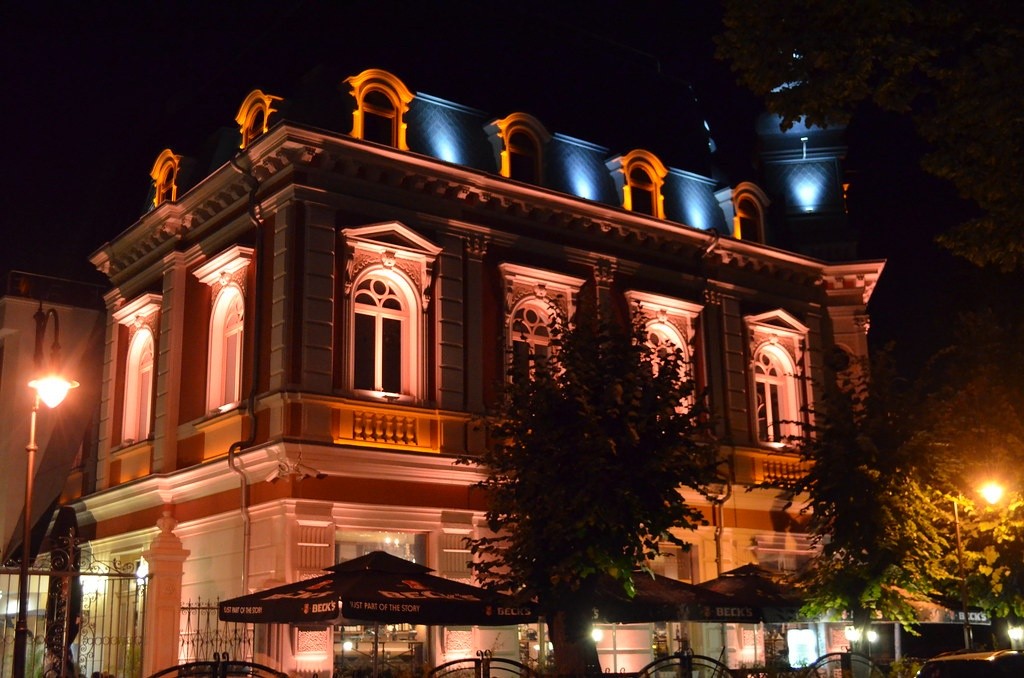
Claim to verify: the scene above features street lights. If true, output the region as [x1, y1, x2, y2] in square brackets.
[11, 293, 79, 677]
[954, 471, 1006, 650]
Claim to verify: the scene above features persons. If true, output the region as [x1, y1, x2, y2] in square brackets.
[44, 648, 81, 678]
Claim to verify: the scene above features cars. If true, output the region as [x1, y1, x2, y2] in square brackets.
[913, 648, 1024, 678]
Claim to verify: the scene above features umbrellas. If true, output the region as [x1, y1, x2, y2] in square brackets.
[218, 550, 539, 678]
[515, 567, 762, 672]
[700, 563, 844, 667]
[818, 578, 992, 678]
